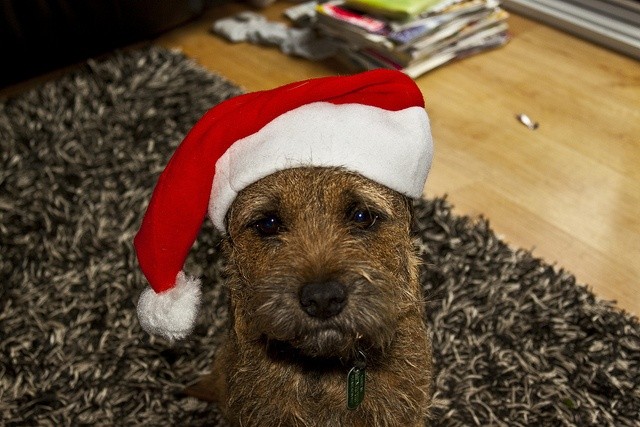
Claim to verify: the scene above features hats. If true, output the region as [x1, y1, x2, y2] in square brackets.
[134, 68, 433, 346]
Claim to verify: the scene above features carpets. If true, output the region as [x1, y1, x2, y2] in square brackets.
[0, 45, 640, 427]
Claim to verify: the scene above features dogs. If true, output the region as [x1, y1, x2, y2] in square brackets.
[136, 68, 433, 427]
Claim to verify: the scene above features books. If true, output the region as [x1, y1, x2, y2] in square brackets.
[281, 0, 511, 82]
